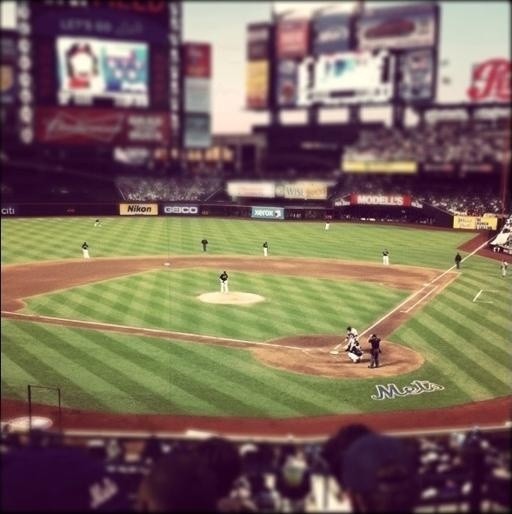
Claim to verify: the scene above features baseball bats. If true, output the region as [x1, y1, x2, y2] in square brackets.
[334, 339, 348, 349]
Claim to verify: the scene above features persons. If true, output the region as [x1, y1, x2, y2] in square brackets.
[367, 333, 381, 367]
[81, 241, 90, 258]
[498, 258, 509, 275]
[454, 252, 461, 267]
[113, 116, 511, 230]
[343, 327, 359, 349]
[94, 218, 102, 226]
[2, 421, 512, 514]
[342, 334, 361, 361]
[262, 240, 269, 256]
[218, 270, 229, 292]
[489, 217, 512, 254]
[382, 248, 390, 266]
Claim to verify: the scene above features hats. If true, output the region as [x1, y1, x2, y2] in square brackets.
[320, 422, 420, 495]
